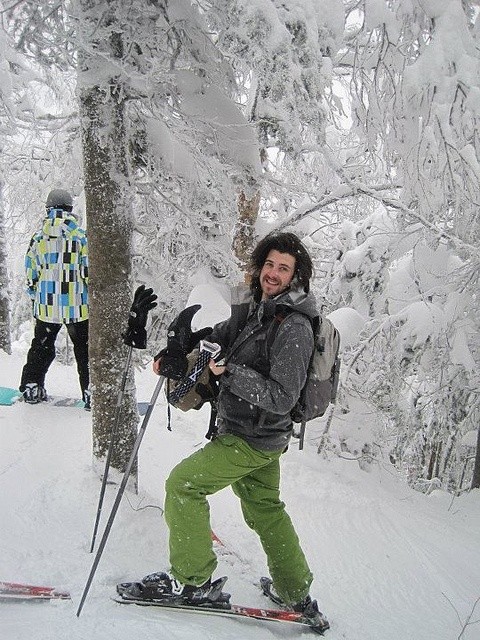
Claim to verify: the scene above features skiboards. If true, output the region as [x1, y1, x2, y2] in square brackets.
[110, 527, 330, 636]
[0, 582, 73, 598]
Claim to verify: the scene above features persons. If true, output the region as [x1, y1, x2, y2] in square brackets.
[117, 232, 313, 607]
[19, 191, 88, 407]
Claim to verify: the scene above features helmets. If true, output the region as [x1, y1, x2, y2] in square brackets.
[45, 189, 72, 209]
[163, 348, 216, 412]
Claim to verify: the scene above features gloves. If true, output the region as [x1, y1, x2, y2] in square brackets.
[155, 304, 213, 382]
[124, 284, 157, 349]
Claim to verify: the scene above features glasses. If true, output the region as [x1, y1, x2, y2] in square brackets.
[199, 338, 216, 357]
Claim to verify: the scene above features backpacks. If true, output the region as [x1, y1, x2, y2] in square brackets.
[263, 309, 340, 423]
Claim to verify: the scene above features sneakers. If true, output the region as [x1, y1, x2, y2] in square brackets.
[142, 571, 213, 607]
[82, 391, 91, 411]
[24, 384, 47, 404]
[268, 582, 311, 613]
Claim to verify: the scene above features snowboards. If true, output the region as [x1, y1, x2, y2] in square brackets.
[0, 387, 150, 416]
